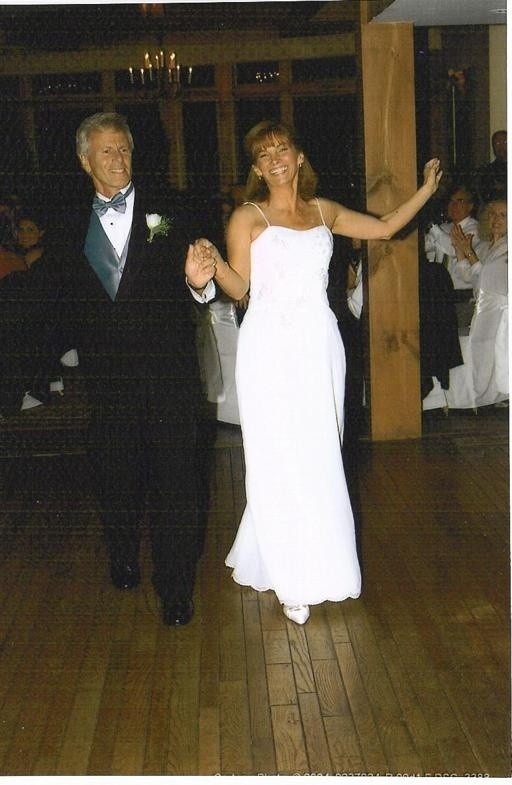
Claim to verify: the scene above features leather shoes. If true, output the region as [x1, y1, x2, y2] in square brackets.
[163, 597, 193, 625]
[282, 603, 310, 625]
[111, 558, 140, 590]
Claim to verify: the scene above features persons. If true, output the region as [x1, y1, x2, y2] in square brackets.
[417, 184, 507, 412]
[2, 112, 219, 628]
[188, 120, 444, 628]
[207, 196, 249, 324]
[330, 236, 366, 408]
[0, 208, 82, 412]
[479, 128, 507, 204]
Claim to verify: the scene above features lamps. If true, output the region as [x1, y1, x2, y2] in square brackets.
[127, 1, 193, 108]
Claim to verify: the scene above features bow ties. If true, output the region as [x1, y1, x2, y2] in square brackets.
[92, 192, 126, 217]
